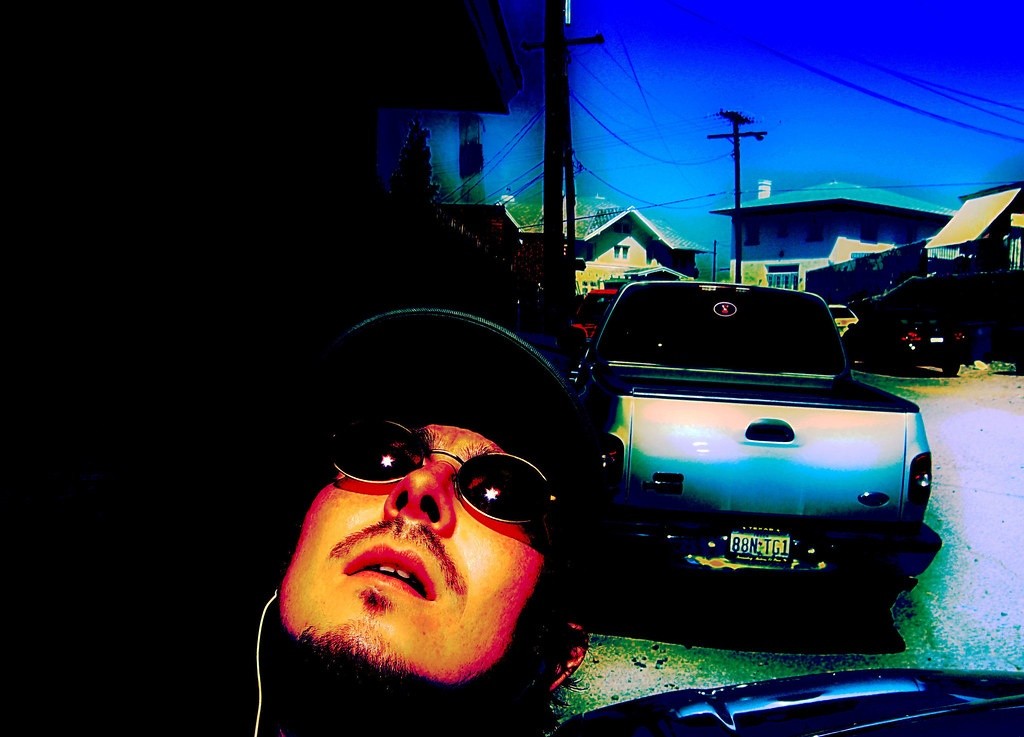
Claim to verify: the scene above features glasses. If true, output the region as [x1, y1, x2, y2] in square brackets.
[327, 414, 558, 550]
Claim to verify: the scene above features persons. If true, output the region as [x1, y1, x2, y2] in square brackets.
[279, 420, 545, 689]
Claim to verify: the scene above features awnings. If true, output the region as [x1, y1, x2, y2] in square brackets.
[924, 188, 1022, 249]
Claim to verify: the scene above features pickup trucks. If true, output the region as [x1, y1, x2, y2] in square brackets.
[564, 281, 932, 587]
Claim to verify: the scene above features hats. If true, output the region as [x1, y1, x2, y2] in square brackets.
[333, 308, 589, 526]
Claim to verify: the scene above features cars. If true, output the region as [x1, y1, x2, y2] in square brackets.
[828, 304, 857, 335]
[842, 309, 971, 377]
[570, 290, 620, 346]
